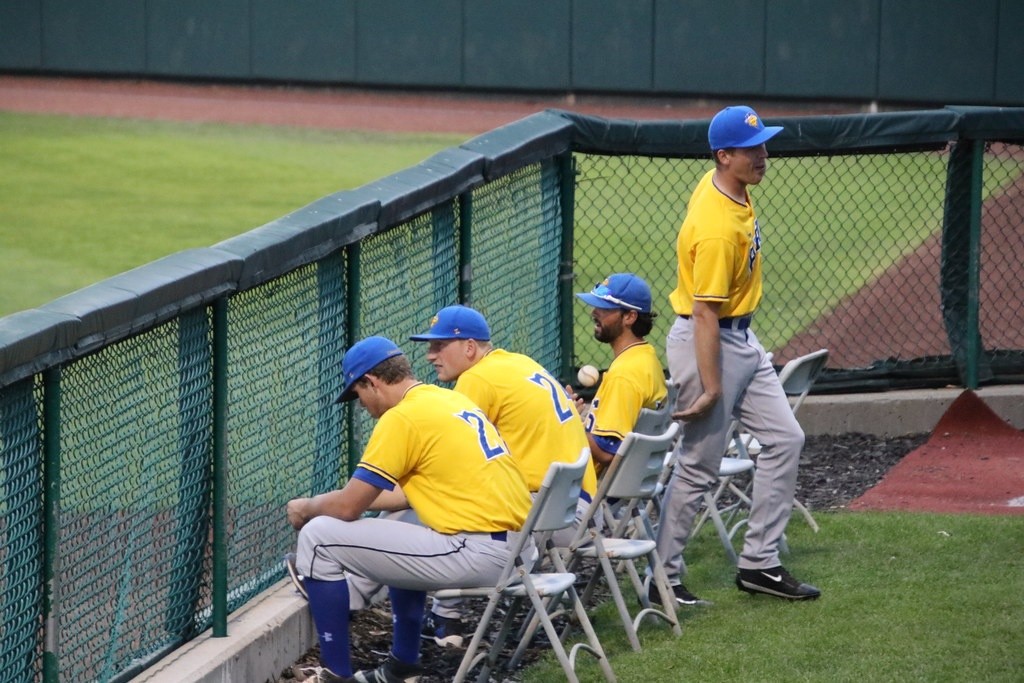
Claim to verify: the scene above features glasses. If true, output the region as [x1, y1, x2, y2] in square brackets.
[591, 281, 642, 310]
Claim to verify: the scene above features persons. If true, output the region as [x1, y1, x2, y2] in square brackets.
[285, 304, 602, 648]
[288, 336, 539, 683]
[566, 271, 667, 479]
[639, 104, 822, 611]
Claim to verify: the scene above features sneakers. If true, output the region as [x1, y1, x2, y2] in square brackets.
[419, 603, 464, 647]
[354, 650, 422, 683]
[647, 581, 699, 605]
[735, 566, 820, 601]
[299, 667, 354, 683]
[285, 552, 309, 600]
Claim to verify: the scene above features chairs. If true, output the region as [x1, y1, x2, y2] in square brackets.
[433, 446, 618, 683]
[506, 348, 830, 674]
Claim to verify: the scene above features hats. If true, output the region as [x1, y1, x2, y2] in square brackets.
[334, 336, 403, 403]
[708, 105, 782, 150]
[408, 305, 490, 341]
[575, 273, 651, 313]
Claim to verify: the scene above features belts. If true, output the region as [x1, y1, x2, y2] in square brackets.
[490, 529, 506, 541]
[679, 314, 752, 331]
[580, 489, 591, 505]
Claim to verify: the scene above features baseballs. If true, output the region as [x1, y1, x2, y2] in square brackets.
[577, 365, 599, 387]
